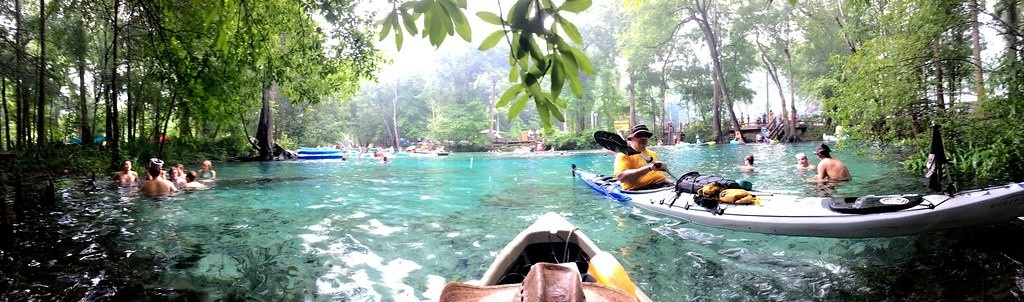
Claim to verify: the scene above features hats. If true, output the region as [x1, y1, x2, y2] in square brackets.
[627, 124, 653, 141]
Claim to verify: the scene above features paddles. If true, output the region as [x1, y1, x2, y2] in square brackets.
[594, 128, 679, 183]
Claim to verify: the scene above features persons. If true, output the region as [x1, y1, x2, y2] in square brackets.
[740, 153, 755, 168]
[197, 160, 216, 179]
[383, 157, 388, 161]
[814, 143, 851, 179]
[140, 166, 178, 193]
[756, 106, 798, 125]
[146, 157, 203, 189]
[752, 125, 779, 144]
[113, 160, 139, 183]
[792, 153, 816, 170]
[613, 124, 676, 190]
[734, 131, 743, 142]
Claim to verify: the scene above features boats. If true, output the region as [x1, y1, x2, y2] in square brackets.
[571, 164, 1024, 239]
[476, 210, 651, 302]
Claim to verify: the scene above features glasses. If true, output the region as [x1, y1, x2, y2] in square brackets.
[634, 132, 650, 138]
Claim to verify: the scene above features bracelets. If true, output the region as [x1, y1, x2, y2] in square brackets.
[649, 167, 651, 171]
